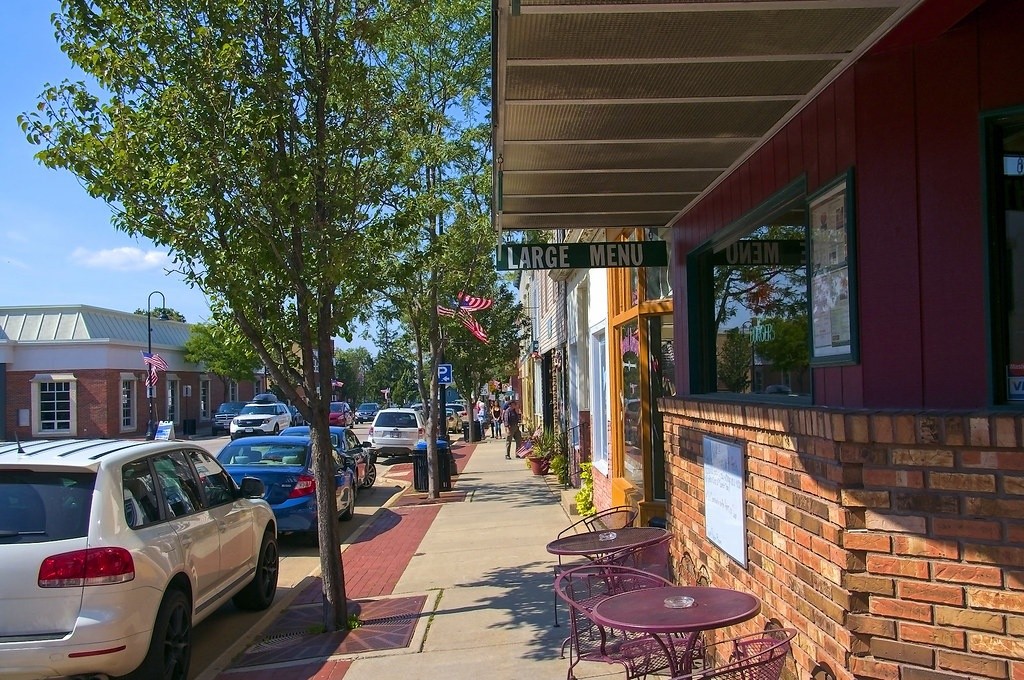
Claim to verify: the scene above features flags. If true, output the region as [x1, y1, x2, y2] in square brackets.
[144, 368, 159, 388]
[437, 303, 455, 317]
[143, 351, 169, 372]
[456, 305, 473, 328]
[452, 290, 492, 312]
[335, 381, 343, 385]
[148, 366, 158, 372]
[464, 318, 490, 346]
[450, 297, 459, 308]
[335, 381, 343, 388]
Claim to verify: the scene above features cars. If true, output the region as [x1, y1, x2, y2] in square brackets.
[354, 403, 381, 424]
[288, 406, 305, 427]
[329, 402, 354, 429]
[410, 403, 469, 434]
[216, 436, 357, 548]
[279, 426, 377, 489]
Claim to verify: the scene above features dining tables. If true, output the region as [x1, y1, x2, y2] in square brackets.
[594, 585, 761, 680]
[546, 527, 672, 590]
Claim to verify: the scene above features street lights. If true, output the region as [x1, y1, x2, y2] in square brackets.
[145, 291, 170, 440]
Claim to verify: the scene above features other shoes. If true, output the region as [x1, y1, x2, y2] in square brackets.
[516, 456, 525, 458]
[496, 436, 502, 439]
[491, 436, 494, 438]
[505, 455, 511, 459]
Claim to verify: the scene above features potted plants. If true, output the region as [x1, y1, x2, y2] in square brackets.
[526, 426, 554, 475]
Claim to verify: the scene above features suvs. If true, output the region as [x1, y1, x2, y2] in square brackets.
[230, 402, 292, 441]
[366, 407, 426, 463]
[211, 402, 250, 436]
[0, 416, 281, 679]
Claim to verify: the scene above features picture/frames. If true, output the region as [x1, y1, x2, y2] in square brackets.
[805, 166, 859, 368]
[702, 435, 748, 570]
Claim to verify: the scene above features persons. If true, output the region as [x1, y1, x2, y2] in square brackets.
[490, 400, 499, 438]
[502, 396, 510, 419]
[505, 400, 526, 459]
[476, 401, 482, 415]
[446, 411, 468, 434]
[483, 401, 489, 429]
[477, 402, 486, 440]
[492, 400, 503, 439]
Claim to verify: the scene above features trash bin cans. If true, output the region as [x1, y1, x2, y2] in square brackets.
[464, 421, 481, 441]
[408, 449, 453, 492]
[414, 439, 450, 448]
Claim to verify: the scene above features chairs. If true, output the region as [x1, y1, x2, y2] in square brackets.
[584, 534, 672, 588]
[669, 628, 797, 680]
[555, 564, 704, 680]
[558, 506, 638, 565]
